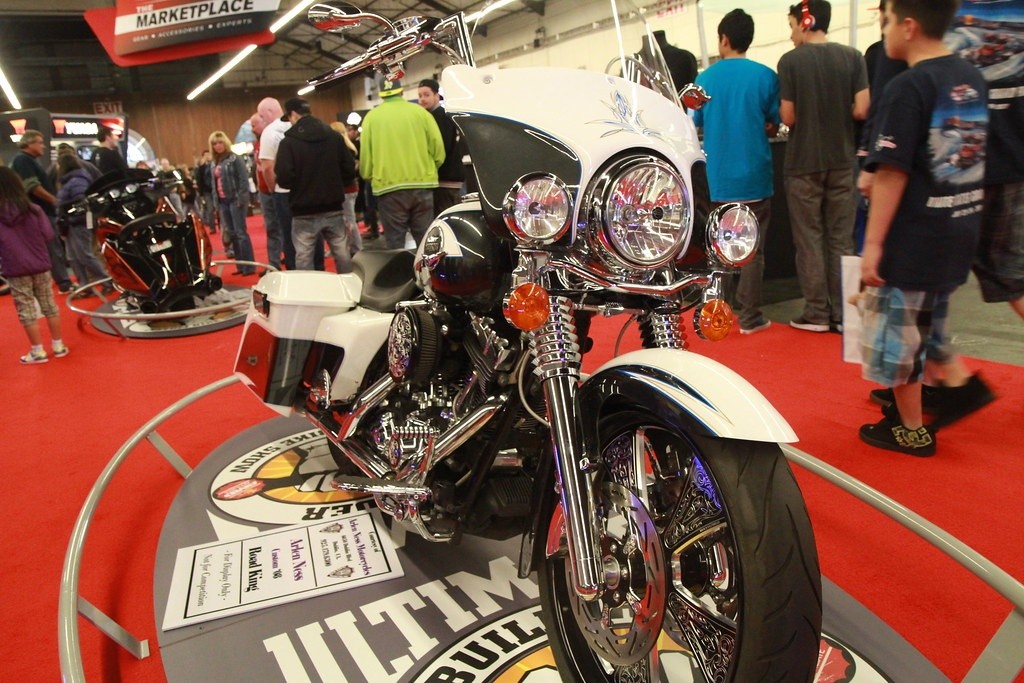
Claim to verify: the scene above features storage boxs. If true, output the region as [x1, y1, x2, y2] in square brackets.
[232, 269, 360, 416]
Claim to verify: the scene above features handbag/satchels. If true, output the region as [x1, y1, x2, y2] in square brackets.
[839, 254, 866, 365]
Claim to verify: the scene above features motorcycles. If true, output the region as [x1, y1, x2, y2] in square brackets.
[64, 143, 223, 321]
[232, 0, 822, 683]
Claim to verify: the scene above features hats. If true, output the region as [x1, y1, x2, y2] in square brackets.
[101, 117, 125, 136]
[419, 79, 444, 101]
[281, 98, 308, 122]
[379, 76, 404, 97]
[346, 112, 362, 125]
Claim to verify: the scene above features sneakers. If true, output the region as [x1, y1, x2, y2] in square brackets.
[790, 317, 830, 331]
[740, 318, 771, 335]
[829, 319, 842, 333]
[860, 411, 936, 458]
[871, 362, 932, 412]
[52, 344, 69, 358]
[927, 372, 995, 432]
[19, 350, 48, 364]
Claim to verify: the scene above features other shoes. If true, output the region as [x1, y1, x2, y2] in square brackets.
[232, 266, 256, 275]
[227, 250, 234, 258]
[258, 266, 282, 277]
[210, 229, 217, 235]
[78, 290, 93, 298]
[101, 285, 114, 295]
[58, 284, 77, 294]
[360, 230, 380, 240]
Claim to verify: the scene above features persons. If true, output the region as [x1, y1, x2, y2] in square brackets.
[89, 128, 127, 172]
[690, 9, 780, 333]
[0, 166, 69, 364]
[857, 0, 1024, 456]
[418, 80, 465, 216]
[136, 97, 380, 275]
[8, 130, 115, 297]
[358, 76, 446, 250]
[777, 0, 870, 332]
